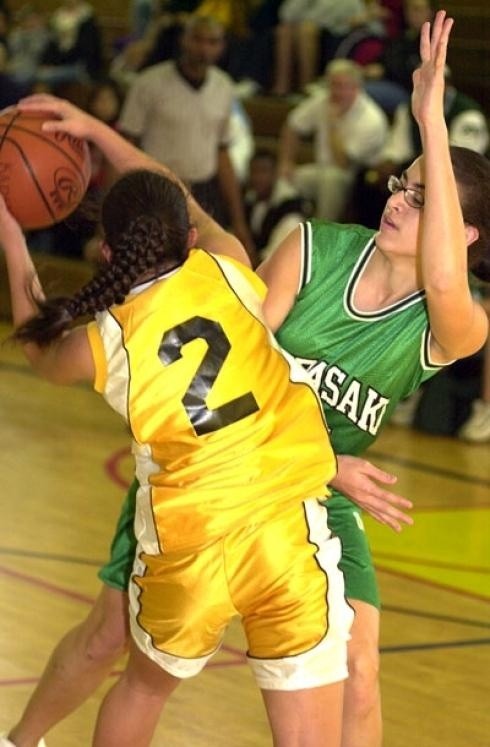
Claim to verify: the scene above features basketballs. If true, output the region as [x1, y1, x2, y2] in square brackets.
[1, 105, 93, 231]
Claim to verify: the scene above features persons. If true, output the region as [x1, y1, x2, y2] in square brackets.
[0, 91, 354, 747]
[1, 10, 490, 747]
[1, 0, 490, 445]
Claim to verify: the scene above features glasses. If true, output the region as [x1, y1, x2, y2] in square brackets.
[386, 174, 425, 209]
